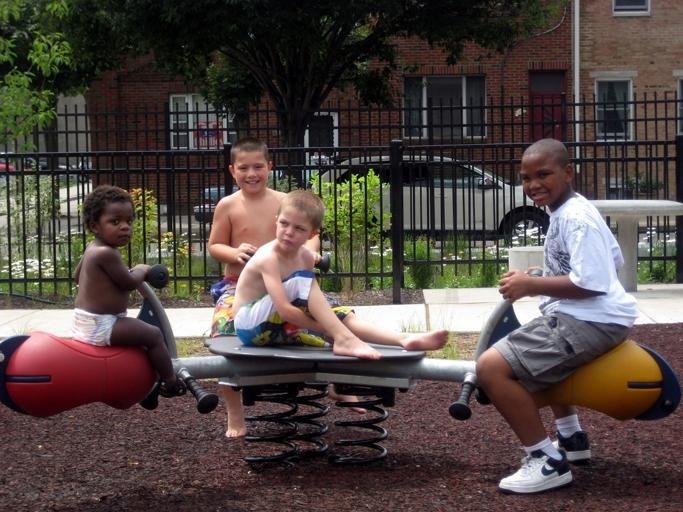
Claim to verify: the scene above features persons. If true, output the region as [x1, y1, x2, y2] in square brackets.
[230, 188, 450, 360]
[70, 183, 190, 399]
[476, 136, 639, 495]
[204, 135, 369, 439]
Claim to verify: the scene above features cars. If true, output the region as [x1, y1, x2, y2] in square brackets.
[303, 155, 553, 247]
[192, 167, 320, 223]
[0, 150, 92, 185]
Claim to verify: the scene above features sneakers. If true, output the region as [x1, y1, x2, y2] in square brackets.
[551, 429, 592, 463]
[496, 449, 574, 496]
